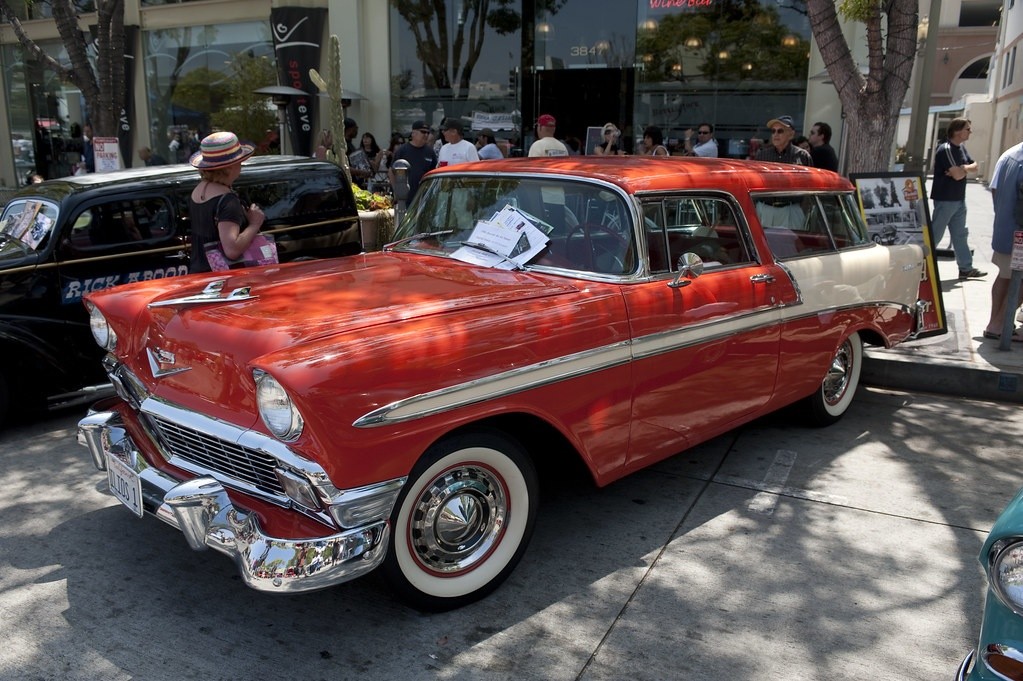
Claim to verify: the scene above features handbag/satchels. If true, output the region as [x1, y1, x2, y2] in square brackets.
[204, 190, 280, 274]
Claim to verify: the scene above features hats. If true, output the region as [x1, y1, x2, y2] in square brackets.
[412, 120, 431, 130]
[189, 130, 256, 171]
[344, 117, 356, 130]
[437, 118, 463, 131]
[476, 128, 493, 136]
[534, 115, 556, 128]
[766, 116, 794, 130]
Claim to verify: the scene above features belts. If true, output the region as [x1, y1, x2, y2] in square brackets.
[760, 200, 800, 208]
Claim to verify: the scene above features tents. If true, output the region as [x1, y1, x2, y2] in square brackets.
[153, 96, 210, 133]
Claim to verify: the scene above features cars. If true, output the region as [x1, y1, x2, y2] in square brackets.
[955, 488, 1023, 681]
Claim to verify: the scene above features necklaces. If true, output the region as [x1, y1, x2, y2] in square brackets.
[201, 178, 228, 199]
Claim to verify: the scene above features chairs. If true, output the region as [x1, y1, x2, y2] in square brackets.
[88, 220, 142, 243]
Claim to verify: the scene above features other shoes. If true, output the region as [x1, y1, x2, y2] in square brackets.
[959, 269, 988, 279]
[984, 330, 1001, 338]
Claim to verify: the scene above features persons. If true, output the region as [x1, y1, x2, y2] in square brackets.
[639, 127, 668, 155]
[428, 116, 505, 233]
[383, 131, 413, 169]
[31, 175, 43, 184]
[389, 120, 438, 206]
[167, 130, 200, 162]
[82, 124, 95, 172]
[188, 132, 265, 273]
[531, 115, 569, 235]
[138, 146, 168, 167]
[313, 118, 383, 187]
[757, 116, 838, 230]
[17, 136, 32, 162]
[593, 123, 627, 155]
[984, 142, 1023, 341]
[684, 123, 718, 157]
[929, 118, 988, 278]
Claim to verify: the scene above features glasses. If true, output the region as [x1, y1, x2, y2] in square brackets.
[770, 128, 792, 134]
[697, 131, 710, 135]
[810, 130, 822, 135]
[415, 130, 430, 136]
[958, 128, 971, 132]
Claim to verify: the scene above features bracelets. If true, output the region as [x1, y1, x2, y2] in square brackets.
[685, 137, 690, 141]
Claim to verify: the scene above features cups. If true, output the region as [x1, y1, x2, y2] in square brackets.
[614, 128, 621, 137]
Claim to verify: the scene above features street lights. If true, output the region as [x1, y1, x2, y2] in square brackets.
[252, 84, 311, 154]
[316, 88, 369, 120]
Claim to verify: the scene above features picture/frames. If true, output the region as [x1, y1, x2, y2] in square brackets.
[849, 170, 947, 340]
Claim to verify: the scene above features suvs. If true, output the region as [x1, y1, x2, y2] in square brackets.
[75, 153, 926, 609]
[0, 154, 364, 425]
